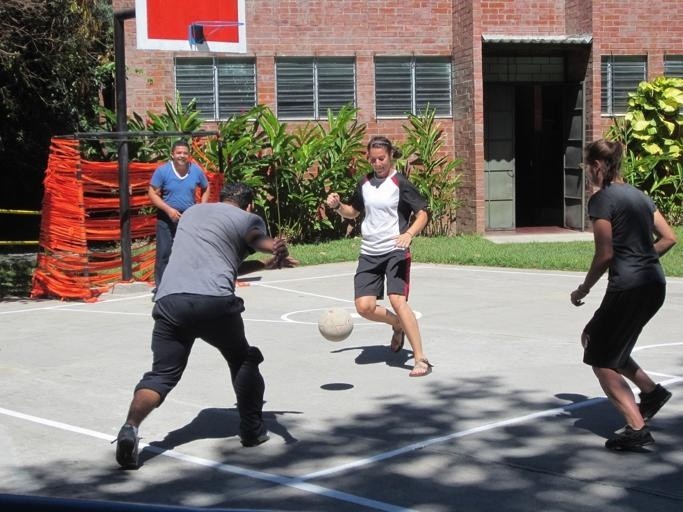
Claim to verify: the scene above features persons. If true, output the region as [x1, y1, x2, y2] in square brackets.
[327, 136, 432, 377]
[569, 139, 676, 451]
[115, 184, 289, 468]
[149, 141, 209, 301]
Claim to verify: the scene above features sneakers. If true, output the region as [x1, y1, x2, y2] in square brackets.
[638, 384, 672, 422]
[116, 423, 138, 467]
[605, 425, 655, 449]
[241, 436, 269, 446]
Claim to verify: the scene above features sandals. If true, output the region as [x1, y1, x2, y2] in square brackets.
[409, 359, 434, 376]
[391, 326, 404, 352]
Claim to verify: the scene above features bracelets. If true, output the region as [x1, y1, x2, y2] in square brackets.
[335, 203, 341, 210]
[578, 284, 589, 293]
[404, 231, 413, 237]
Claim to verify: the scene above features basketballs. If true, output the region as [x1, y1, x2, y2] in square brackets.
[319, 307, 354, 341]
